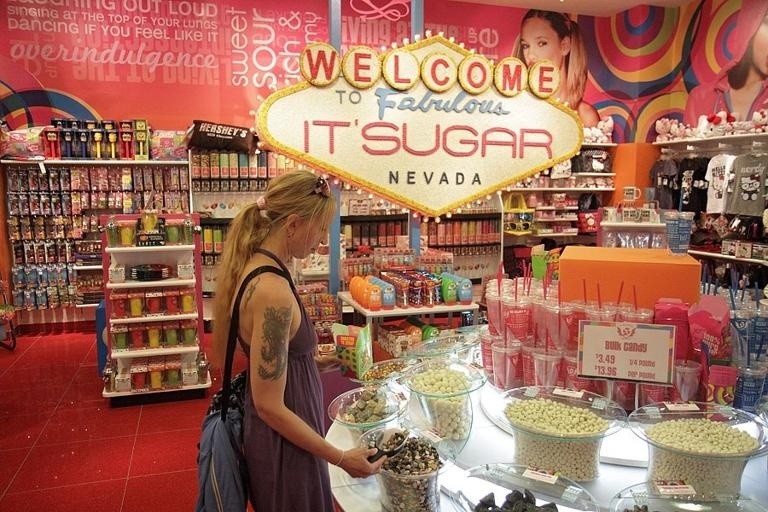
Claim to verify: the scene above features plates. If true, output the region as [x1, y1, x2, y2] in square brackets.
[128, 263, 173, 282]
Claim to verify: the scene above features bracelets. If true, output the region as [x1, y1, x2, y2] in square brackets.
[334, 449, 345, 467]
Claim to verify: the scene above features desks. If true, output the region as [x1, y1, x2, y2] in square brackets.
[317, 323, 767, 512]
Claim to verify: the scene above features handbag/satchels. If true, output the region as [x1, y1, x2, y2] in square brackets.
[206, 368, 247, 422]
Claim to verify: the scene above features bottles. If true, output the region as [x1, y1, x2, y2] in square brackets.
[198, 361, 208, 384]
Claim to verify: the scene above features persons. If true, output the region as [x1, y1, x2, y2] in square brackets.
[512, 9, 605, 129]
[681, 0, 768, 128]
[210, 167, 388, 512]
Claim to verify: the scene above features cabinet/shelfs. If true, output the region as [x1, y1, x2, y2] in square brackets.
[96, 207, 216, 397]
[190, 127, 618, 290]
[336, 289, 477, 385]
[645, 125, 768, 304]
[1, 128, 191, 321]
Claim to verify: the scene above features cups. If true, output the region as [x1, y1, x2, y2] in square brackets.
[146, 297, 161, 313]
[664, 211, 696, 258]
[131, 372, 146, 388]
[183, 327, 196, 345]
[150, 371, 165, 388]
[165, 329, 177, 345]
[111, 332, 127, 349]
[113, 299, 126, 317]
[132, 331, 145, 348]
[182, 294, 195, 314]
[167, 369, 178, 386]
[104, 212, 193, 247]
[129, 297, 145, 318]
[148, 328, 162, 347]
[166, 295, 177, 315]
[103, 368, 116, 393]
[478, 276, 767, 422]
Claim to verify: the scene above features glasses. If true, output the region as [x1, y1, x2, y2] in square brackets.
[308, 174, 332, 198]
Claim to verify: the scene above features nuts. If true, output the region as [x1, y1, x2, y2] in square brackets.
[644, 417, 760, 498]
[474, 488, 558, 512]
[504, 396, 606, 483]
[623, 503, 651, 512]
[340, 358, 473, 512]
